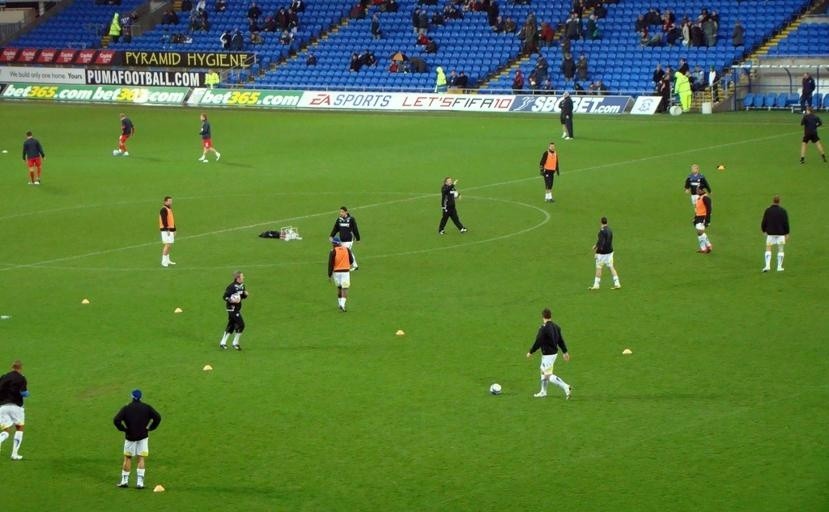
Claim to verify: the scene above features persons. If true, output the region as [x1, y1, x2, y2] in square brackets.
[540, 143, 560, 203]
[526, 309, 572, 400]
[328, 237, 358, 311]
[160, 197, 176, 267]
[328, 207, 360, 272]
[438, 177, 467, 235]
[693, 185, 712, 253]
[22, 131, 44, 184]
[113, 391, 161, 489]
[761, 195, 790, 272]
[0, 360, 30, 461]
[589, 218, 621, 290]
[219, 271, 248, 351]
[685, 165, 710, 204]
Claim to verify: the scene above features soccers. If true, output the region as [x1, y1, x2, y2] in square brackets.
[231, 294, 239, 303]
[112, 150, 120, 156]
[489, 383, 501, 394]
[696, 223, 704, 230]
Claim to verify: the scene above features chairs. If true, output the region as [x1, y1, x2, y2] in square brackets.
[7, 2, 143, 50]
[744, 91, 828, 111]
[105, 1, 828, 91]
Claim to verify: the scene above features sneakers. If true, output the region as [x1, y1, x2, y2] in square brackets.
[763, 267, 770, 273]
[533, 391, 548, 398]
[198, 151, 221, 162]
[220, 344, 229, 350]
[588, 286, 600, 290]
[695, 243, 712, 254]
[117, 481, 129, 487]
[610, 284, 620, 290]
[232, 344, 241, 352]
[10, 453, 24, 460]
[565, 384, 572, 400]
[439, 228, 468, 236]
[777, 267, 785, 272]
[338, 305, 347, 312]
[161, 261, 177, 267]
[136, 482, 145, 489]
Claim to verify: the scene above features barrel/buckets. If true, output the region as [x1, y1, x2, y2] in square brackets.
[701, 101, 712, 114]
[669, 105, 683, 116]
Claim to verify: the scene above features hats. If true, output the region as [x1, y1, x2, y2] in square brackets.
[332, 237, 341, 245]
[132, 389, 142, 400]
